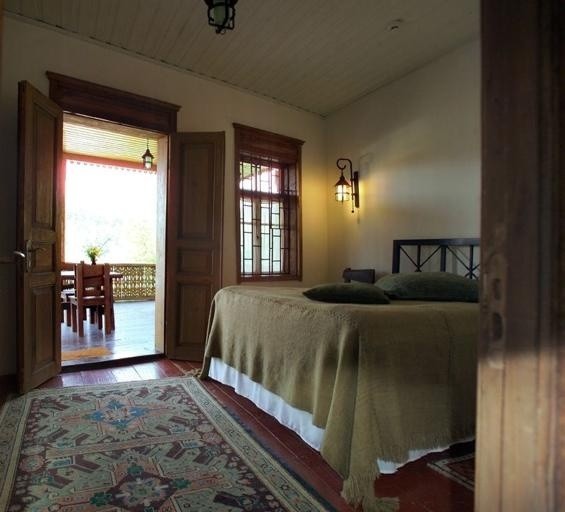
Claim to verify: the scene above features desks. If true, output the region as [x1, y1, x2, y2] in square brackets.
[61, 270, 123, 331]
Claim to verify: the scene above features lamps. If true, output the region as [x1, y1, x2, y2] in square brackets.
[333, 158, 359, 213]
[141, 138, 154, 170]
[204, 0, 238, 35]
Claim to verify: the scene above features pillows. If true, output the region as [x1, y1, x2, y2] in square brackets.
[302, 271, 480, 305]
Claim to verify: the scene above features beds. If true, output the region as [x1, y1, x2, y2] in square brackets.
[209, 237, 480, 476]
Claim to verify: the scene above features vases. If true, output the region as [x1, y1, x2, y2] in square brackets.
[91, 259, 96, 265]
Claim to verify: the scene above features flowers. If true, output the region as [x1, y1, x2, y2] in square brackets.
[83, 238, 110, 261]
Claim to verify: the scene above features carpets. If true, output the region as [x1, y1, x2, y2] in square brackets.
[426, 452, 475, 492]
[2, 376, 341, 512]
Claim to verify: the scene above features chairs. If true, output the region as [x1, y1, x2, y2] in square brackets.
[68, 263, 111, 337]
[61, 258, 84, 326]
